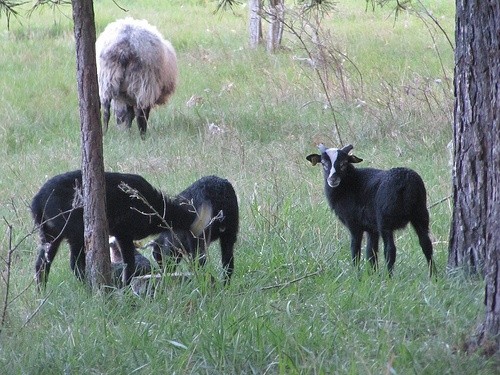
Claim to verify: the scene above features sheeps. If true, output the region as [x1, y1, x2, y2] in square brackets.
[306, 142, 435, 278]
[95, 17, 179, 141]
[31, 172, 239, 293]
